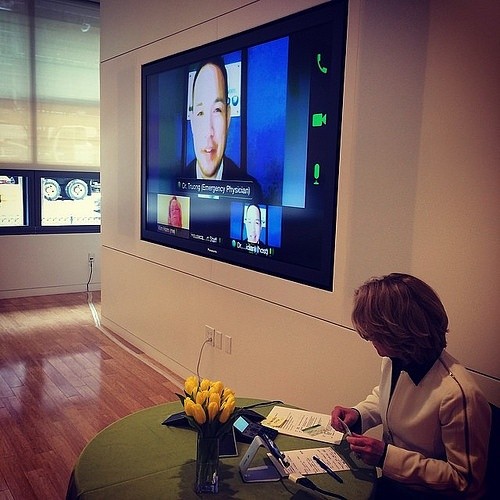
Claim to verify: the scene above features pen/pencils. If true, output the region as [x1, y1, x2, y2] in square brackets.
[312, 455, 345, 483]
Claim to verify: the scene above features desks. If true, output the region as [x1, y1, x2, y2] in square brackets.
[66, 395, 397, 500]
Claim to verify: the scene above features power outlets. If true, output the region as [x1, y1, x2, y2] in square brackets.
[88, 252, 94, 263]
[203, 325, 215, 347]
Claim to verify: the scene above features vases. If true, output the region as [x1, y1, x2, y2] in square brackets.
[196, 431, 221, 496]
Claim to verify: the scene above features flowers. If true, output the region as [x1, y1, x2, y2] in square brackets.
[161, 377, 285, 439]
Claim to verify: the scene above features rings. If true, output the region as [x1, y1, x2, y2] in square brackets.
[355, 451, 361, 459]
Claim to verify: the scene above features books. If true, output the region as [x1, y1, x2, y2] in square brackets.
[259, 405, 344, 446]
[271, 446, 350, 479]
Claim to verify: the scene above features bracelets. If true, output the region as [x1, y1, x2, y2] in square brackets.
[351, 408, 360, 417]
[375, 443, 388, 469]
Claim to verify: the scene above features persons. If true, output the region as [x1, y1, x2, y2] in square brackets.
[331, 273, 492, 500]
[245, 202, 265, 246]
[182, 62, 262, 201]
[168, 196, 183, 230]
